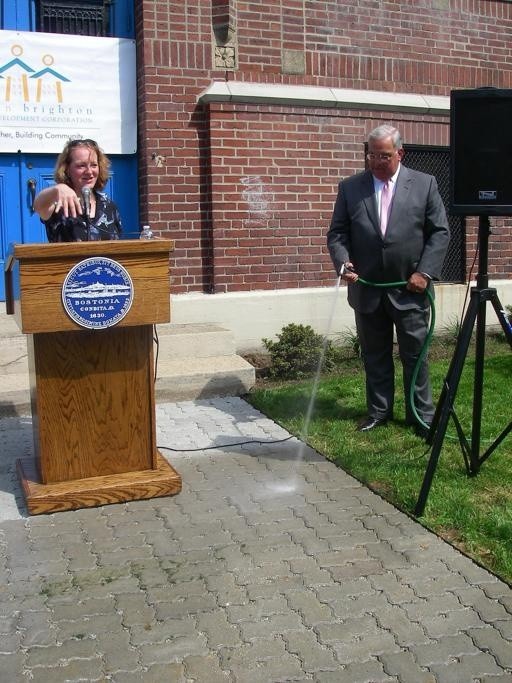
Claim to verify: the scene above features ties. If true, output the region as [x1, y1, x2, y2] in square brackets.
[380, 181, 388, 237]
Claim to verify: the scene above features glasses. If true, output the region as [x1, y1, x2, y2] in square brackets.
[367, 153, 389, 161]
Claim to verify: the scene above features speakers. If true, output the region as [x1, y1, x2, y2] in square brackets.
[449, 87, 512, 216]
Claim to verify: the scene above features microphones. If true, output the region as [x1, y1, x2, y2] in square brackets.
[80, 186, 91, 243]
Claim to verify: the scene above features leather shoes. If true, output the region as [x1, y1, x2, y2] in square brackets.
[360, 417, 382, 431]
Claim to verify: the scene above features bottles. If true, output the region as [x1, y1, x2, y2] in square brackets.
[140, 226, 153, 240]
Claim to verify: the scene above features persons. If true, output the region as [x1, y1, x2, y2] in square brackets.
[326, 125, 452, 442]
[33, 139, 125, 242]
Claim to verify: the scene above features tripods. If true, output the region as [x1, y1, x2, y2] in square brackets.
[411, 216, 512, 516]
